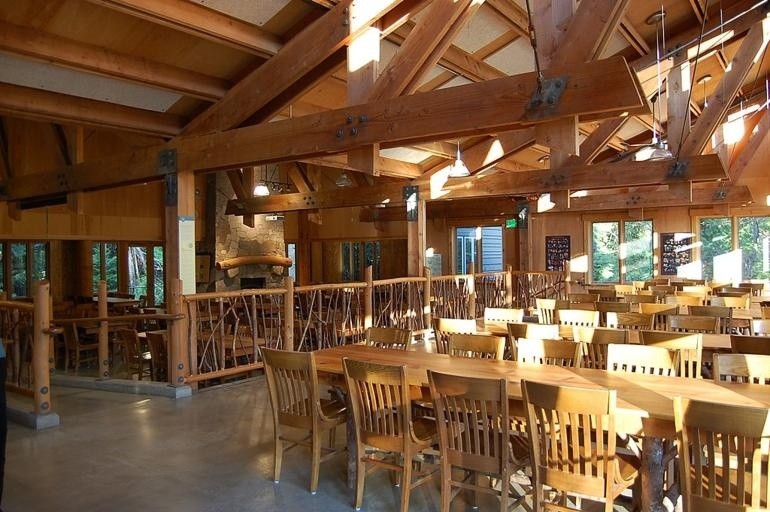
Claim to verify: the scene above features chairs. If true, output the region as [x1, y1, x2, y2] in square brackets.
[4, 290, 284, 390]
